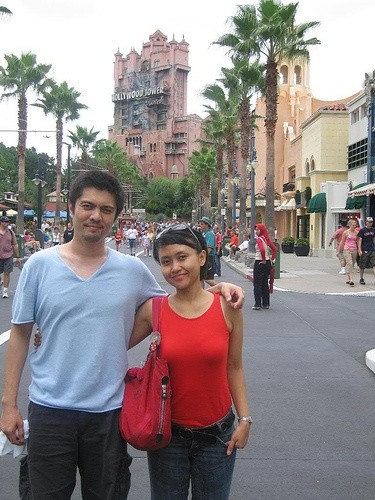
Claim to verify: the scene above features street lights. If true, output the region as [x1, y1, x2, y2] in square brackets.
[230, 160, 239, 229]
[220, 178, 226, 249]
[32, 155, 47, 249]
[246, 127, 260, 267]
[61, 143, 71, 221]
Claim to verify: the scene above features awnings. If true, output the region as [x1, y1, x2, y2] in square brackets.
[344, 183, 369, 210]
[274, 197, 296, 211]
[347, 184, 375, 197]
[307, 193, 326, 213]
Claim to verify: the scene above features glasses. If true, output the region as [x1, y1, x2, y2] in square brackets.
[154, 222, 199, 243]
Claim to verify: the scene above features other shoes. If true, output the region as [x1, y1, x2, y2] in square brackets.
[252, 306, 260, 310]
[346, 281, 354, 285]
[338, 267, 346, 275]
[360, 278, 365, 284]
[261, 306, 269, 309]
[2, 288, 8, 298]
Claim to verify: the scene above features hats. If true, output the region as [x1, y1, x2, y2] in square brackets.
[199, 217, 214, 228]
[366, 217, 373, 223]
[0, 216, 11, 222]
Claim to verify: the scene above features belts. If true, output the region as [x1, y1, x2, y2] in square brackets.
[169, 408, 235, 437]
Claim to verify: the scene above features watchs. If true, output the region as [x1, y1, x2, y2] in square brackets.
[238, 416, 253, 422]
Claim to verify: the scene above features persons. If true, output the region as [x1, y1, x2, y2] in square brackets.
[32, 173, 42, 185]
[198, 217, 215, 280]
[0, 169, 245, 500]
[0, 216, 20, 298]
[274, 228, 277, 243]
[214, 224, 253, 263]
[32, 222, 250, 500]
[114, 219, 204, 256]
[328, 216, 375, 285]
[251, 224, 277, 310]
[8, 218, 73, 253]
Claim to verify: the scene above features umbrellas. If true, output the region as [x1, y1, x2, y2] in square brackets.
[0, 210, 73, 218]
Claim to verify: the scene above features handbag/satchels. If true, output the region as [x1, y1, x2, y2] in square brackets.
[119, 296, 173, 451]
